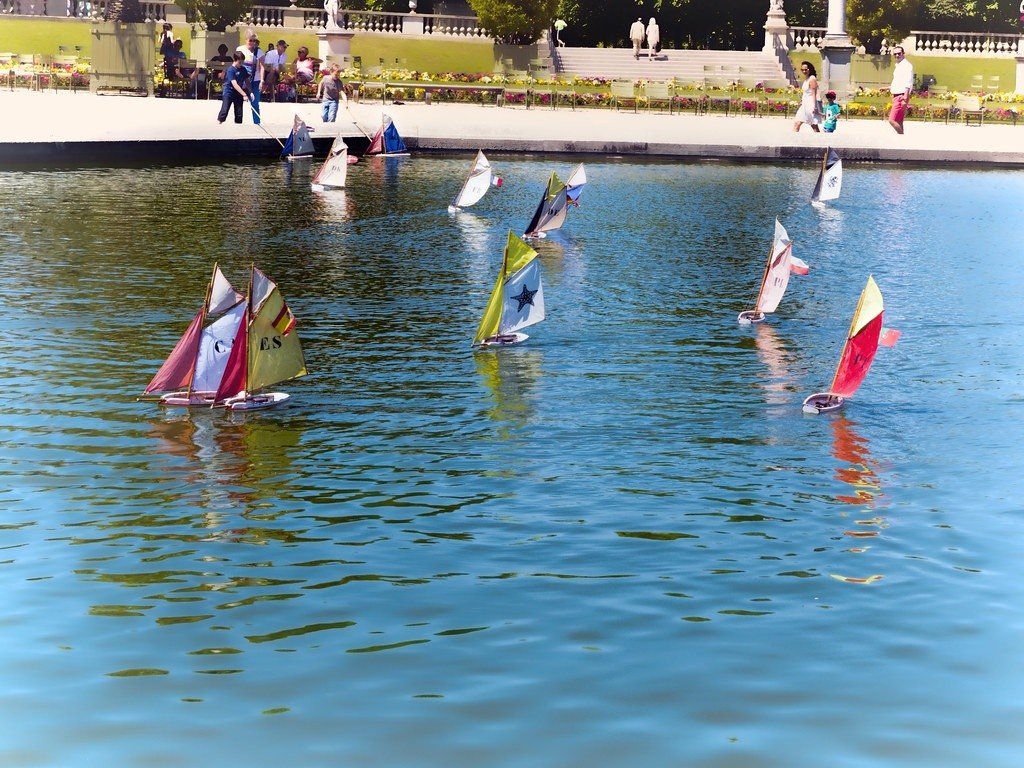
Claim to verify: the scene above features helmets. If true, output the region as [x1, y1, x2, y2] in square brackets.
[825, 91, 836, 97]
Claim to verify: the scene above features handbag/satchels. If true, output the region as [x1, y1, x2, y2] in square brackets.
[243, 62, 255, 84]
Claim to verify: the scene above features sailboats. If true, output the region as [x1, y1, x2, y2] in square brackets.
[448, 148, 503, 212]
[811, 147, 843, 211]
[364, 113, 411, 159]
[803, 271, 900, 414]
[737, 216, 810, 325]
[137, 259, 252, 405]
[311, 132, 349, 190]
[470, 228, 546, 345]
[279, 113, 314, 162]
[522, 161, 588, 241]
[212, 260, 309, 412]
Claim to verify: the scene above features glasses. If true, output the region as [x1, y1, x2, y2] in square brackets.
[827, 96, 834, 99]
[893, 52, 901, 55]
[801, 69, 809, 72]
[298, 51, 301, 54]
[248, 39, 255, 42]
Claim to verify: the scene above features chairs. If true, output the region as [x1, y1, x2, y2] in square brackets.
[0, 52, 79, 94]
[163, 55, 386, 106]
[502, 77, 792, 119]
[923, 85, 983, 127]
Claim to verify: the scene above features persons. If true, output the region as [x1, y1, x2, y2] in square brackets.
[316, 63, 349, 122]
[814, 91, 841, 132]
[209, 44, 234, 80]
[217, 51, 255, 124]
[256, 39, 262, 49]
[265, 44, 274, 55]
[794, 61, 823, 133]
[285, 47, 323, 101]
[158, 23, 168, 44]
[880, 46, 914, 134]
[629, 18, 645, 60]
[265, 42, 288, 102]
[235, 28, 266, 125]
[155, 24, 180, 67]
[646, 17, 660, 61]
[554, 17, 570, 47]
[166, 40, 199, 80]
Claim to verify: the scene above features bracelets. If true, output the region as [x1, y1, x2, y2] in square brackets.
[260, 80, 264, 84]
[243, 95, 247, 96]
